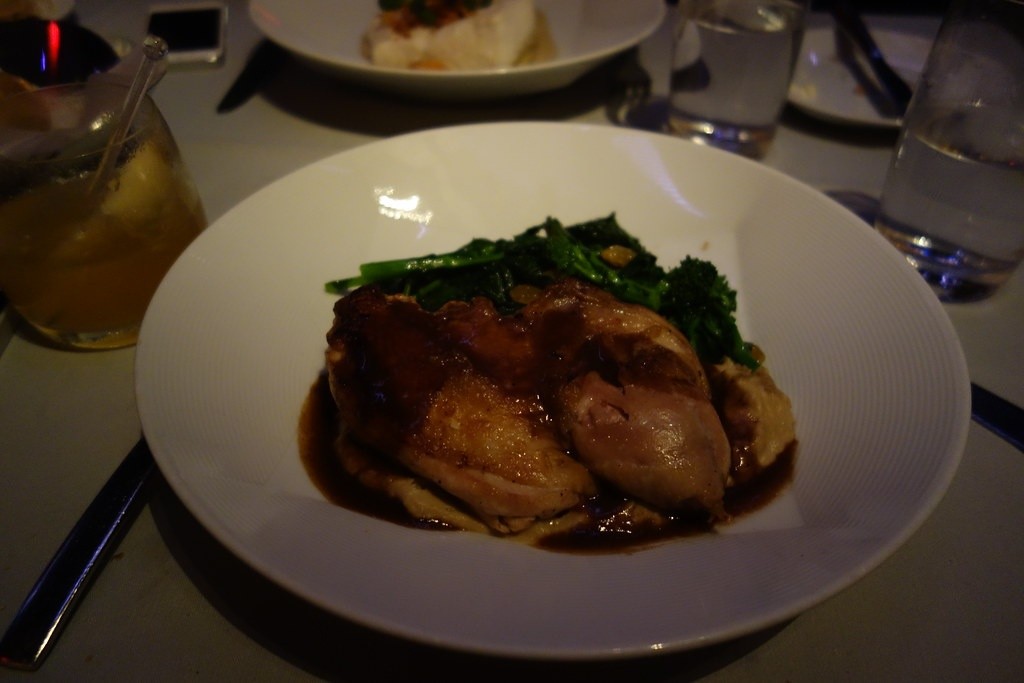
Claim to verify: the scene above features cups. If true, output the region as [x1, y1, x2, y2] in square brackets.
[664, 0, 811, 158]
[878, 0, 1024, 299]
[0, 81, 207, 349]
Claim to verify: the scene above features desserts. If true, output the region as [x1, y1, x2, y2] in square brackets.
[361, 0, 556, 71]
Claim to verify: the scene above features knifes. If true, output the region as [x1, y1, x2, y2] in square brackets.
[827, 0, 910, 109]
[218, 37, 278, 111]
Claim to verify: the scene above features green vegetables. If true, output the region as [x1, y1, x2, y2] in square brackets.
[324, 209, 761, 371]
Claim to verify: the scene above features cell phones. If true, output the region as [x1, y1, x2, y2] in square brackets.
[148, 0, 228, 63]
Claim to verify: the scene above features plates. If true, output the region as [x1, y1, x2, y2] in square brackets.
[787, 25, 932, 129]
[247, 0, 668, 104]
[136, 121, 970, 662]
